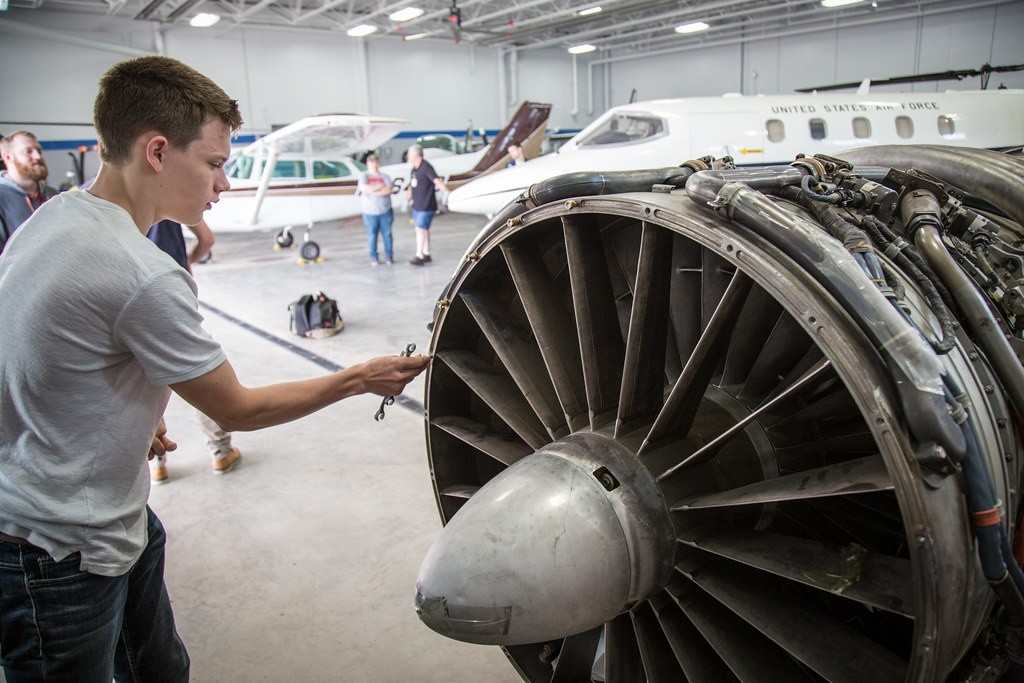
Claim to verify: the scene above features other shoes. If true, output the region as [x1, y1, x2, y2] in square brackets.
[410, 257, 423, 265]
[424, 253, 431, 262]
[386, 257, 394, 264]
[371, 260, 381, 266]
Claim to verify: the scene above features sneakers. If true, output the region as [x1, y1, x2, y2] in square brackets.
[213, 446, 242, 475]
[150, 466, 169, 485]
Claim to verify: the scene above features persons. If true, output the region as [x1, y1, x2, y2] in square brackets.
[146, 218, 243, 486]
[358, 155, 394, 266]
[0, 132, 59, 257]
[406, 144, 449, 265]
[0, 59, 430, 683]
[505, 142, 530, 168]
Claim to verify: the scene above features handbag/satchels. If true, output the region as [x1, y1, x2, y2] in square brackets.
[289, 291, 345, 339]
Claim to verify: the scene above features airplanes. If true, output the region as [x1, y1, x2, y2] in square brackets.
[445, 77, 1023, 216]
[180, 101, 552, 261]
